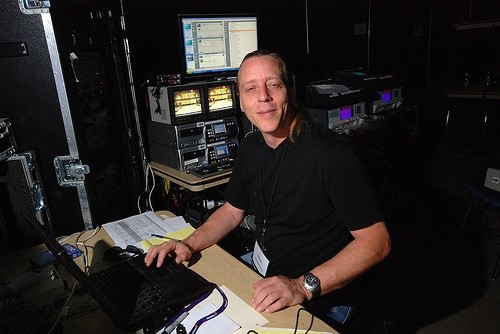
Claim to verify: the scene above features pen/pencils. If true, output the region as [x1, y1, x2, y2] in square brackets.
[161, 312, 188, 334]
[151, 233, 172, 240]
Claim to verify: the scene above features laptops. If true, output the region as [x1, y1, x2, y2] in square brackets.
[24, 216, 215, 332]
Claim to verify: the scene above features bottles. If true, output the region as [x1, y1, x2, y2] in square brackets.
[483, 71, 497, 91]
[464, 73, 472, 92]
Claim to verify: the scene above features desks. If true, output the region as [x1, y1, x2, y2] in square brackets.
[0, 209, 342, 334]
[149, 159, 234, 219]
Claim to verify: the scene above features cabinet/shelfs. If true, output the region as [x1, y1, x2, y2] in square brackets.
[441, 17, 500, 100]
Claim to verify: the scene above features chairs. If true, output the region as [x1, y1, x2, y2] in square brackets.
[459, 184, 500, 282]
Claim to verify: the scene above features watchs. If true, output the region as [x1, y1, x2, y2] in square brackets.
[302, 273, 321, 300]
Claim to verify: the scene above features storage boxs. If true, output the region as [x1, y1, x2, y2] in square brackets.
[484, 167, 500, 192]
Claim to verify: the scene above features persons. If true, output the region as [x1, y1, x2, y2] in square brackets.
[145, 49, 391, 334]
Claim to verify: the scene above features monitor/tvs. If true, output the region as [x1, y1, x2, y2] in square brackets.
[177, 14, 262, 81]
[169, 83, 237, 119]
